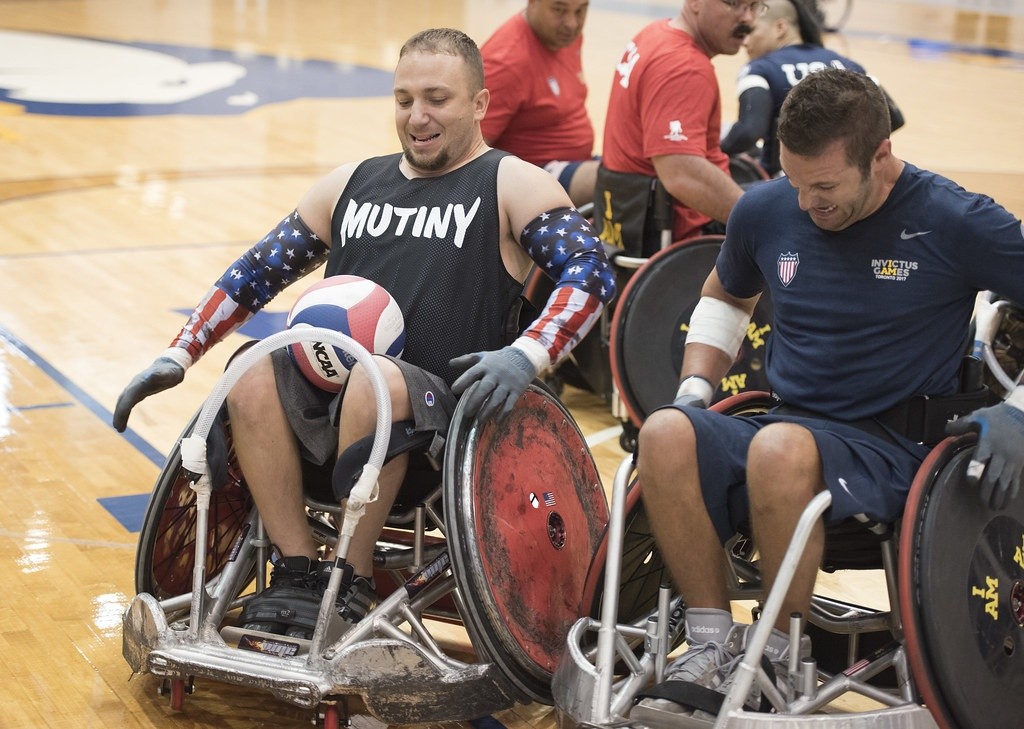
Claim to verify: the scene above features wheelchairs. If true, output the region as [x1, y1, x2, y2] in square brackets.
[121, 326, 611, 729]
[521, 168, 775, 449]
[552, 392, 1024, 729]
[729, 157, 770, 189]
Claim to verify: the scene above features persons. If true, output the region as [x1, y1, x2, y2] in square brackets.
[719, 0, 904, 180]
[113, 28, 617, 630]
[628, 68, 1024, 724]
[477, 0, 603, 209]
[592, 0, 750, 247]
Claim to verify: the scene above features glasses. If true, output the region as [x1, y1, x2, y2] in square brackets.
[721, 0, 769, 17]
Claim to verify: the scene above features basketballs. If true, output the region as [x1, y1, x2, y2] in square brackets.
[287, 274, 408, 396]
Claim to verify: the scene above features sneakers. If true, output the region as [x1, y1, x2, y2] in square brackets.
[692, 620, 812, 723]
[244, 542, 377, 657]
[640, 608, 749, 714]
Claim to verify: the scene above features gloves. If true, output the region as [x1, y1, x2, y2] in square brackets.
[113, 356, 186, 434]
[450, 346, 540, 427]
[944, 400, 1024, 511]
